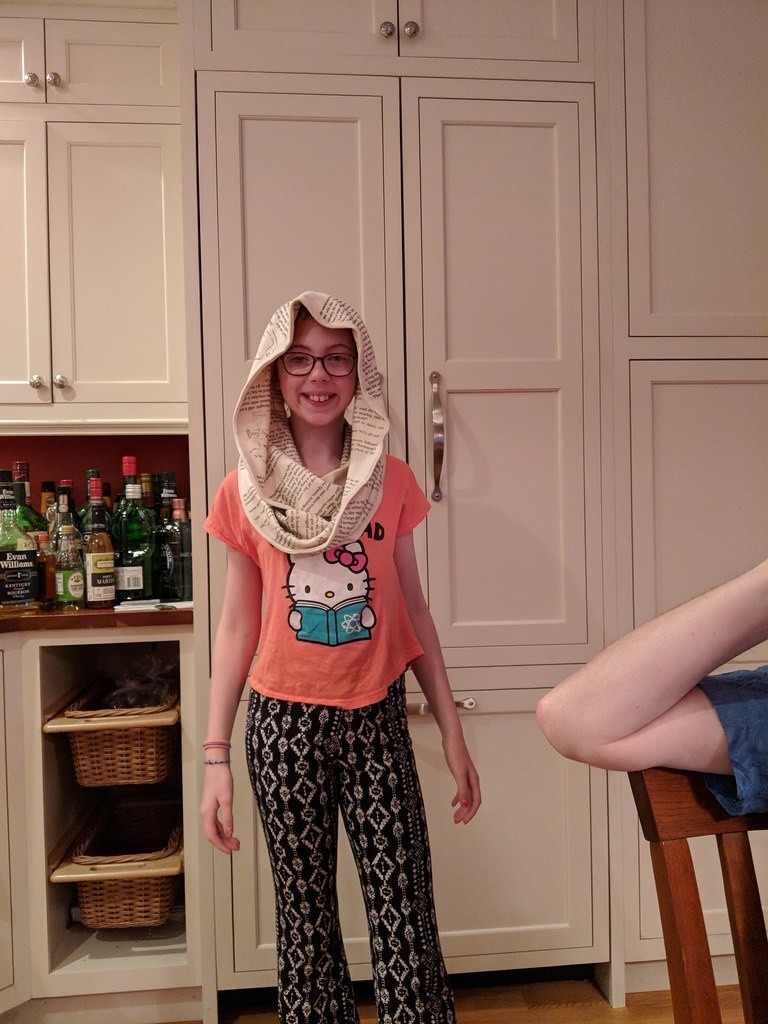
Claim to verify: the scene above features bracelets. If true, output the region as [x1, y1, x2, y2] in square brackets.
[205, 758, 230, 767]
[203, 740, 232, 750]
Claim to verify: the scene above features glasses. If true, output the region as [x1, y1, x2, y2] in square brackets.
[280, 352, 357, 378]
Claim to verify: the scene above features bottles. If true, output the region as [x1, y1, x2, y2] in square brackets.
[0, 455, 194, 616]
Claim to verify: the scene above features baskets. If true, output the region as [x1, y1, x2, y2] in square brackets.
[73, 800, 182, 927]
[64, 674, 176, 786]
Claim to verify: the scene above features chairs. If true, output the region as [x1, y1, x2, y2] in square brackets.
[627, 766, 768, 1024]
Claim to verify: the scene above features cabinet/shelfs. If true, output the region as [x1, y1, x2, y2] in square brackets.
[0, 0, 189, 438]
[193, 67, 603, 668]
[0, 623, 219, 1024]
[209, 1, 579, 62]
[232, 676, 594, 974]
[582, 1, 768, 1010]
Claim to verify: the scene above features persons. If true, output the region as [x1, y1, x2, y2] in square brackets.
[535, 555, 768, 821]
[199, 291, 485, 1024]
